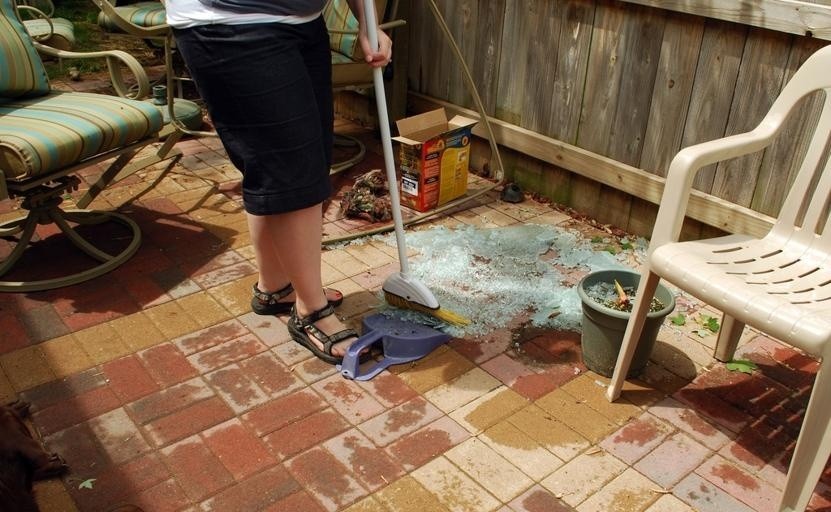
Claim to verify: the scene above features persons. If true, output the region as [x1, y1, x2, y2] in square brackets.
[157, 0, 395, 364]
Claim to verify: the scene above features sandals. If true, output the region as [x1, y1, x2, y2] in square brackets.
[251, 281, 372, 366]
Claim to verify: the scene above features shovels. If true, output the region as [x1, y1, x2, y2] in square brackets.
[336, 310, 449, 381]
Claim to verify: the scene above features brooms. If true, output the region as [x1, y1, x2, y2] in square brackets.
[363, 0, 471, 328]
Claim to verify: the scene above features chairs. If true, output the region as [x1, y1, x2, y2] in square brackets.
[322, 1, 399, 174]
[15, 0, 76, 72]
[607, 43, 831, 512]
[0, 0, 164, 293]
[92, 0, 177, 98]
